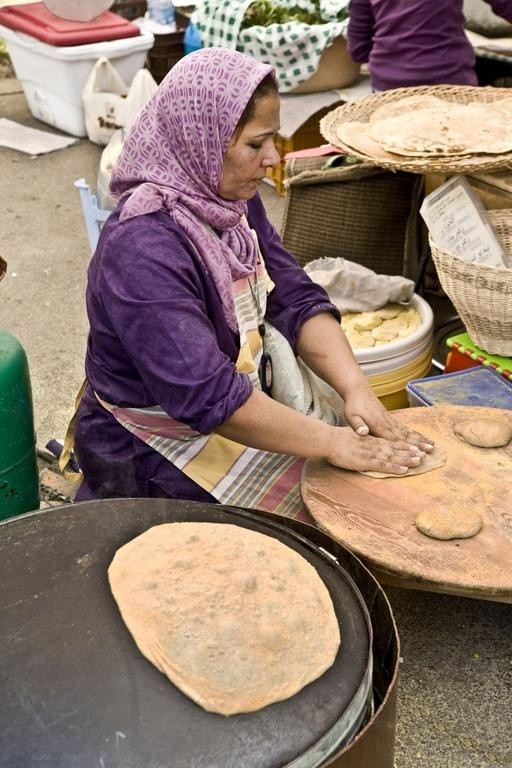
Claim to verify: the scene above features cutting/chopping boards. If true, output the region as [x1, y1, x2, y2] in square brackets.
[301, 405, 512, 598]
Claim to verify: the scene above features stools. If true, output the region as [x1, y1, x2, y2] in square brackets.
[74, 177, 112, 249]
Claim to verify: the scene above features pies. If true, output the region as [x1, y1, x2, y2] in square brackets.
[336, 93, 512, 163]
[105, 523, 343, 716]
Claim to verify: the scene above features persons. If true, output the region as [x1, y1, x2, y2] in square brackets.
[73, 48, 434, 502]
[347, 0, 512, 93]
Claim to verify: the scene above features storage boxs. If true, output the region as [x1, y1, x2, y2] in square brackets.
[0, 25, 155, 137]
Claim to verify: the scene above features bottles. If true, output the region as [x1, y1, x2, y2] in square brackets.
[146, 0, 177, 32]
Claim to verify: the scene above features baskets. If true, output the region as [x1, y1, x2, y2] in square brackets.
[428, 208, 512, 359]
